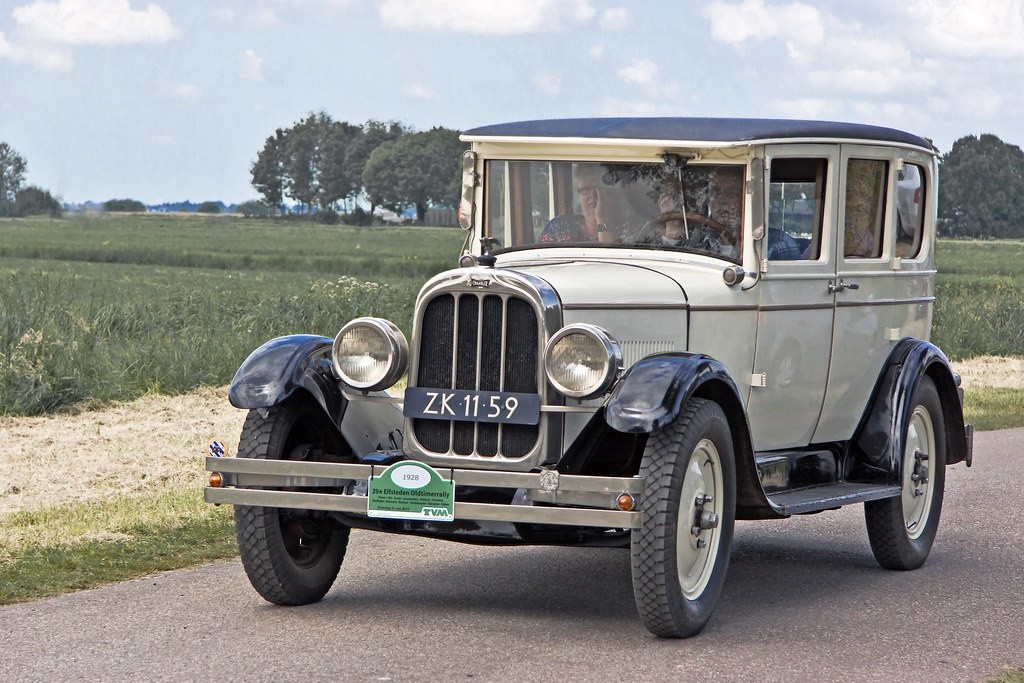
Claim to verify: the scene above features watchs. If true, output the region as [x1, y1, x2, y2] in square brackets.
[595, 220, 617, 233]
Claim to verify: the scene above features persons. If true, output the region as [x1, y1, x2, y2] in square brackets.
[537, 163, 646, 248]
[659, 166, 802, 261]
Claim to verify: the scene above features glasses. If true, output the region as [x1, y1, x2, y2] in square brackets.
[577, 186, 591, 196]
[708, 188, 742, 198]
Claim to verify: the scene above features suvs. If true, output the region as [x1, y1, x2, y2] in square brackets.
[202, 117, 974, 640]
